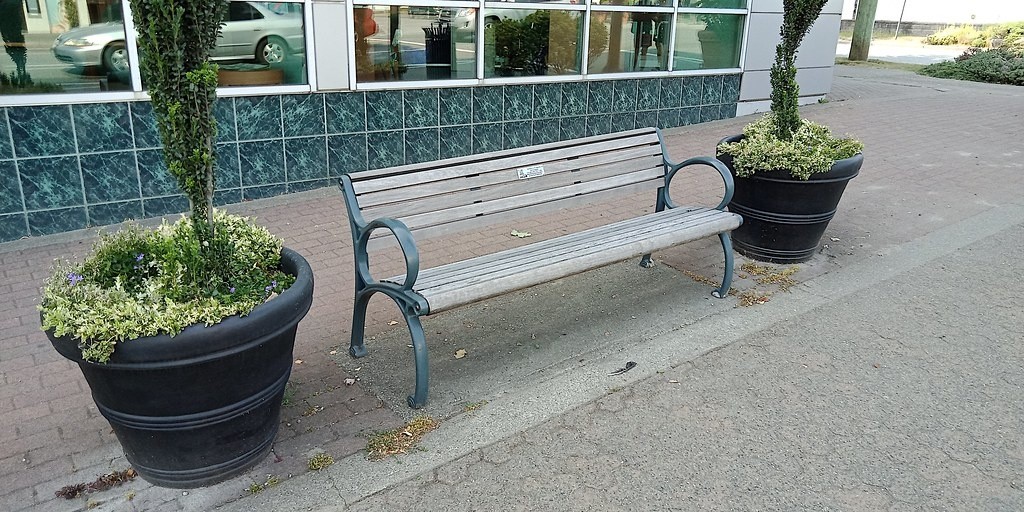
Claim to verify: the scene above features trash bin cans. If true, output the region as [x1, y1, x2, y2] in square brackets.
[421, 21, 457, 79]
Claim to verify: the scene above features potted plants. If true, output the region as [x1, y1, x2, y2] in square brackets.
[691, 0, 742, 69]
[714, 0, 864, 266]
[37, 0, 315, 491]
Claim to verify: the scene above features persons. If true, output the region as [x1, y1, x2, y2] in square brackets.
[631, 0, 670, 70]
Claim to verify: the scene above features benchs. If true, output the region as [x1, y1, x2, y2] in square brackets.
[338, 127, 743, 410]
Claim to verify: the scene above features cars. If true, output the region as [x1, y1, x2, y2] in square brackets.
[354, 7, 377, 41]
[436, 0, 584, 46]
[50, 1, 306, 82]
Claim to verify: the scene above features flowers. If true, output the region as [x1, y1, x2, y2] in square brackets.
[717, 112, 865, 181]
[32, 206, 297, 367]
